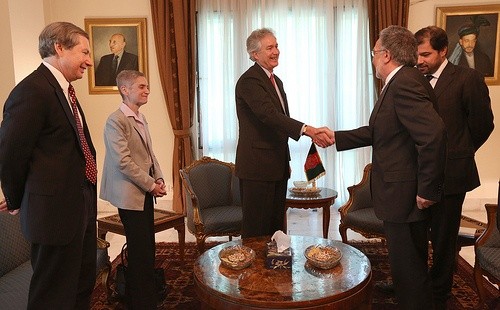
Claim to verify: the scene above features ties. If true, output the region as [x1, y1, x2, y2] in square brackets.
[112, 55, 118, 74]
[68, 83, 97, 185]
[424, 75, 434, 82]
[270, 74, 277, 92]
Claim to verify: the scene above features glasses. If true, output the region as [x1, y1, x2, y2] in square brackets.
[371, 49, 386, 56]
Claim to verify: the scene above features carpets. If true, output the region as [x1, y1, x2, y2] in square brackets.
[86, 240, 500, 310]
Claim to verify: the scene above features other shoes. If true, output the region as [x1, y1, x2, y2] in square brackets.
[376, 283, 394, 294]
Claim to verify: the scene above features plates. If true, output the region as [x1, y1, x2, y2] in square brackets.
[288, 187, 322, 194]
[218, 245, 256, 270]
[304, 243, 343, 269]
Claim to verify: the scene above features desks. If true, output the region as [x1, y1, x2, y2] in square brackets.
[192, 235, 372, 310]
[283, 186, 339, 239]
[97, 208, 186, 262]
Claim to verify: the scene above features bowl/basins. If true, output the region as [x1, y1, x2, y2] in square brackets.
[293, 181, 308, 188]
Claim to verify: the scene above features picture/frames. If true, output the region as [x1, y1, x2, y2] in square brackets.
[82, 17, 150, 97]
[434, 3, 500, 86]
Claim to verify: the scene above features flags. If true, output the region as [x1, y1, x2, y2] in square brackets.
[303, 142, 326, 184]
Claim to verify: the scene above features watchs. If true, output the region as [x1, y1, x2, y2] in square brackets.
[302, 125, 308, 136]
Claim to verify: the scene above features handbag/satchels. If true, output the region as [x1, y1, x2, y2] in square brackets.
[117, 243, 165, 305]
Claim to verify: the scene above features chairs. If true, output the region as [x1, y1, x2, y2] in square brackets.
[0, 198, 33, 310]
[473, 203, 500, 310]
[178, 156, 243, 255]
[338, 163, 386, 247]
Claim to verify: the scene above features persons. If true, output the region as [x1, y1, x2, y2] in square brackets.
[94, 34, 139, 86]
[313, 25, 439, 310]
[411, 26, 493, 310]
[0, 21, 96, 310]
[447, 25, 494, 77]
[234, 28, 332, 239]
[98, 70, 167, 271]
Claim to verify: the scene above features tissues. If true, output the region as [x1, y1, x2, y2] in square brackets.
[265, 229, 292, 270]
